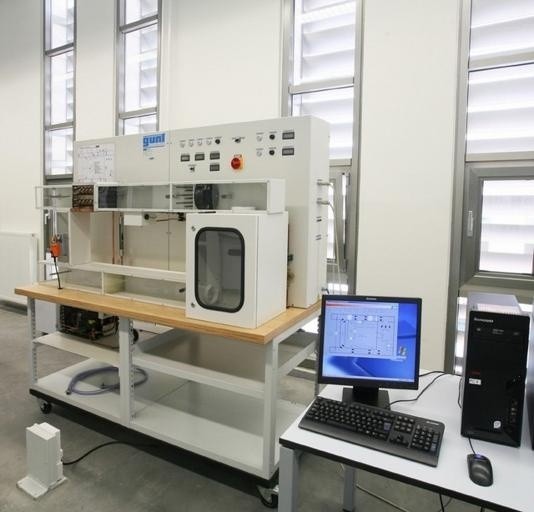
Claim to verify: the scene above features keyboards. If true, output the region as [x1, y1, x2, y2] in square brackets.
[298, 396, 444, 468]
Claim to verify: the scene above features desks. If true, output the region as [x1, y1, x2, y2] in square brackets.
[274, 368, 532, 512]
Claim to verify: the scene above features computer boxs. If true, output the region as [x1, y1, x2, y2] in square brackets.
[460, 291, 530, 448]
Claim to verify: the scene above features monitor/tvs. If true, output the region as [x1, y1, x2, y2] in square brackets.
[316, 293, 421, 411]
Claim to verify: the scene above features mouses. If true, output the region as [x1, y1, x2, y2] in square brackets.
[468, 453, 492, 487]
